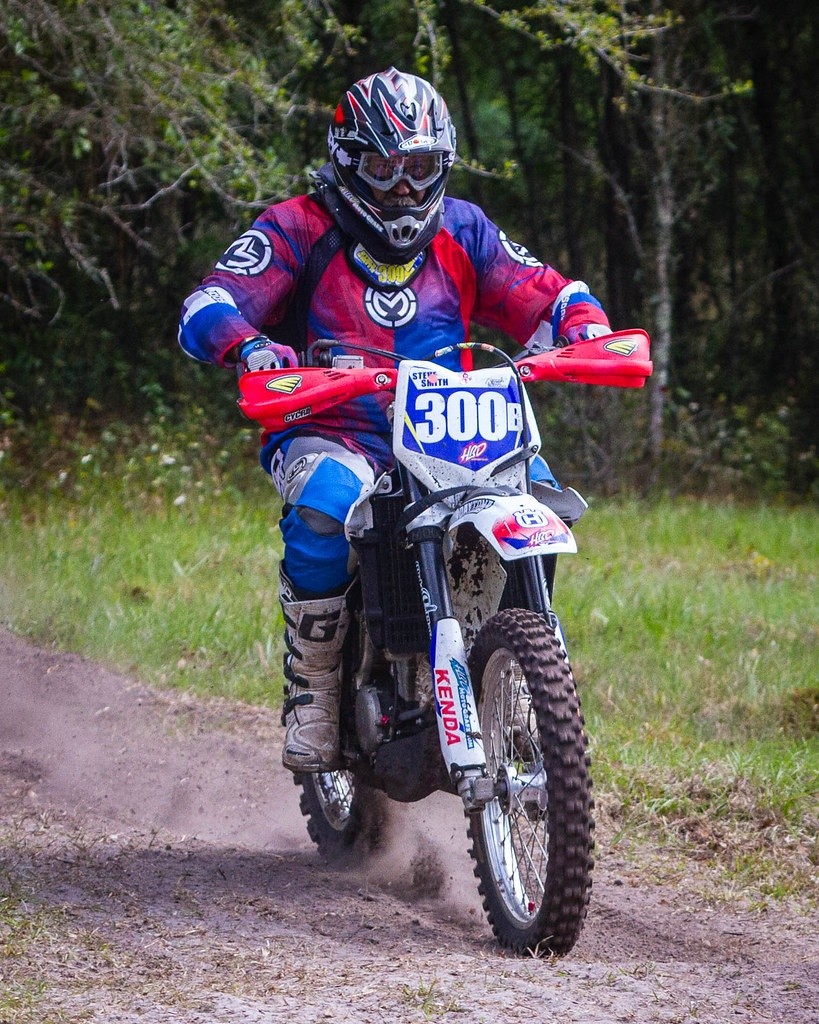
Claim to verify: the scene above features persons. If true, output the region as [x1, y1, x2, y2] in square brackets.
[175, 66, 615, 774]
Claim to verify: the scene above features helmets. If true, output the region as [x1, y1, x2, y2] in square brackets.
[327, 66, 457, 249]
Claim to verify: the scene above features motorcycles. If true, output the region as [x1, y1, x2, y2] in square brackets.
[238, 327, 655, 959]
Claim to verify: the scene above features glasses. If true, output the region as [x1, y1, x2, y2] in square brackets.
[352, 150, 444, 193]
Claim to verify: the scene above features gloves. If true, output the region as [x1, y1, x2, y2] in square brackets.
[566, 323, 613, 344]
[237, 334, 299, 372]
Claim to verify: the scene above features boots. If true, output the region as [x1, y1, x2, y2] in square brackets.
[509, 661, 541, 762]
[278, 560, 358, 774]
